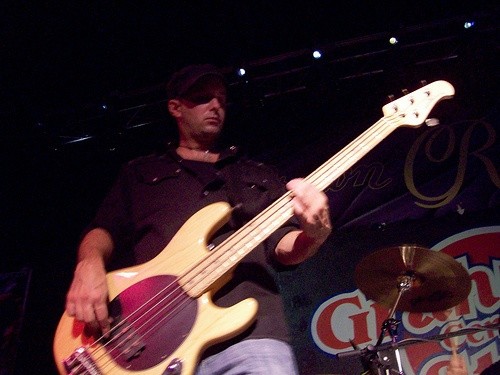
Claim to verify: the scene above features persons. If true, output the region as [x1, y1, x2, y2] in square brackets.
[65, 60, 332, 375]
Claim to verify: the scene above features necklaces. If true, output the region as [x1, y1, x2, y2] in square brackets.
[179, 144, 210, 154]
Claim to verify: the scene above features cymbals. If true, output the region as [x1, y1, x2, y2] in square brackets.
[354, 245, 473, 313]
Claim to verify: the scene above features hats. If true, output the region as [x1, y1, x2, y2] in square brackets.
[166, 63, 224, 99]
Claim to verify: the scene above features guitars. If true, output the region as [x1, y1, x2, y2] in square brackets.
[54, 80, 456, 375]
